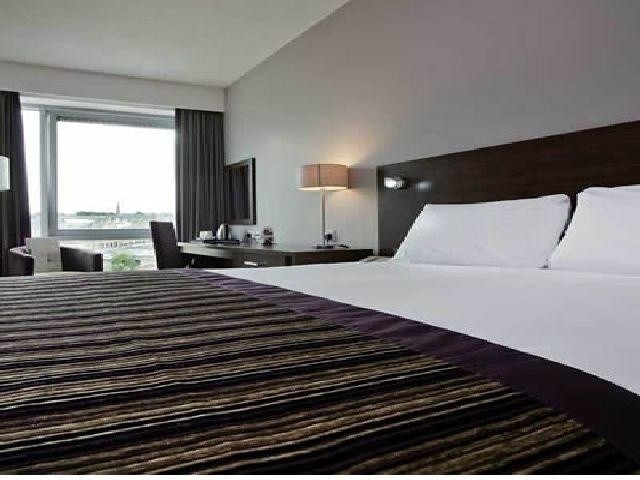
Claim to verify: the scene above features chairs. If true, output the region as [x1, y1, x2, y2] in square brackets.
[10, 245, 104, 276]
[150, 222, 198, 269]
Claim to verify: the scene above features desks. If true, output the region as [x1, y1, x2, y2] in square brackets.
[177, 241, 374, 269]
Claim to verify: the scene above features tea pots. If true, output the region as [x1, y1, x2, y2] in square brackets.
[218, 223, 232, 240]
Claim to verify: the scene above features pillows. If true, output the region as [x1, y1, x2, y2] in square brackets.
[24, 236, 64, 273]
[395, 194, 570, 271]
[546, 186, 640, 277]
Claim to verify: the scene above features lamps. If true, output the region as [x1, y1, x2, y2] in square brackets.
[300, 164, 350, 249]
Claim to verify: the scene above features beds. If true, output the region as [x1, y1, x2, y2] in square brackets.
[0, 120, 640, 475]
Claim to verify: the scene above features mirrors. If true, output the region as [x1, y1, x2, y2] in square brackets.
[223, 158, 256, 226]
